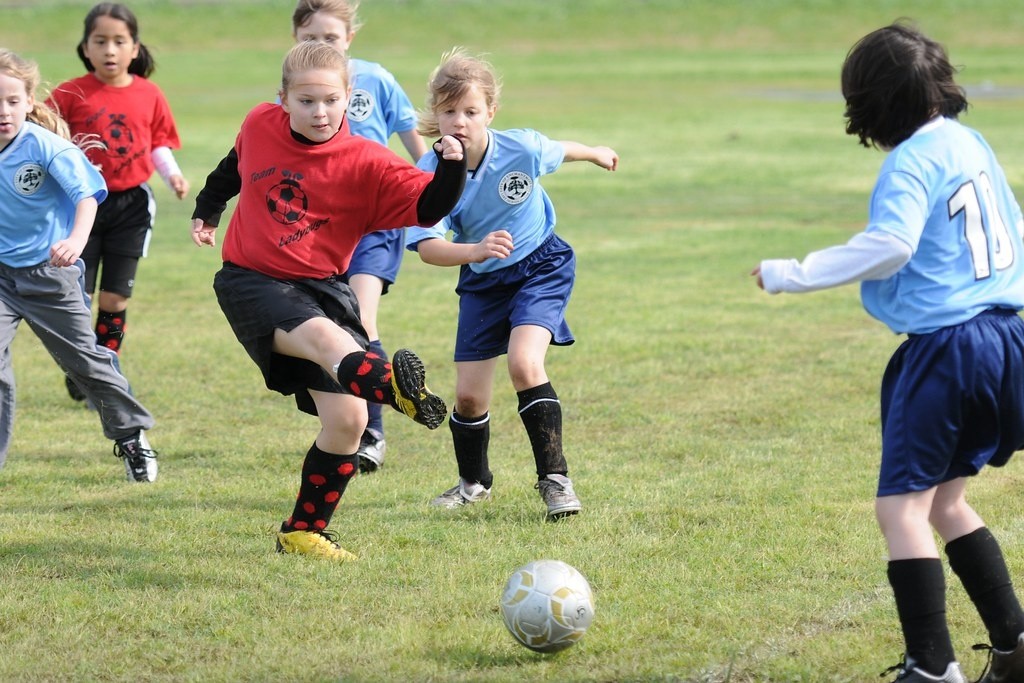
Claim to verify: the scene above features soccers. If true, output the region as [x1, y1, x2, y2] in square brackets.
[504, 561, 593, 651]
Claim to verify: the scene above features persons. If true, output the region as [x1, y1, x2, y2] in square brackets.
[404, 56, 618, 522]
[191, 43, 467, 560]
[0, 49, 158, 482]
[43, 2, 190, 410]
[751, 26, 1024, 683]
[278, 0, 428, 475]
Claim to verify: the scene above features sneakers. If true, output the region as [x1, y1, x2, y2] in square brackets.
[115, 428, 158, 485]
[435, 478, 492, 508]
[536, 472, 581, 522]
[356, 427, 388, 473]
[891, 660, 965, 683]
[392, 348, 448, 430]
[972, 632, 1023, 683]
[275, 522, 357, 562]
